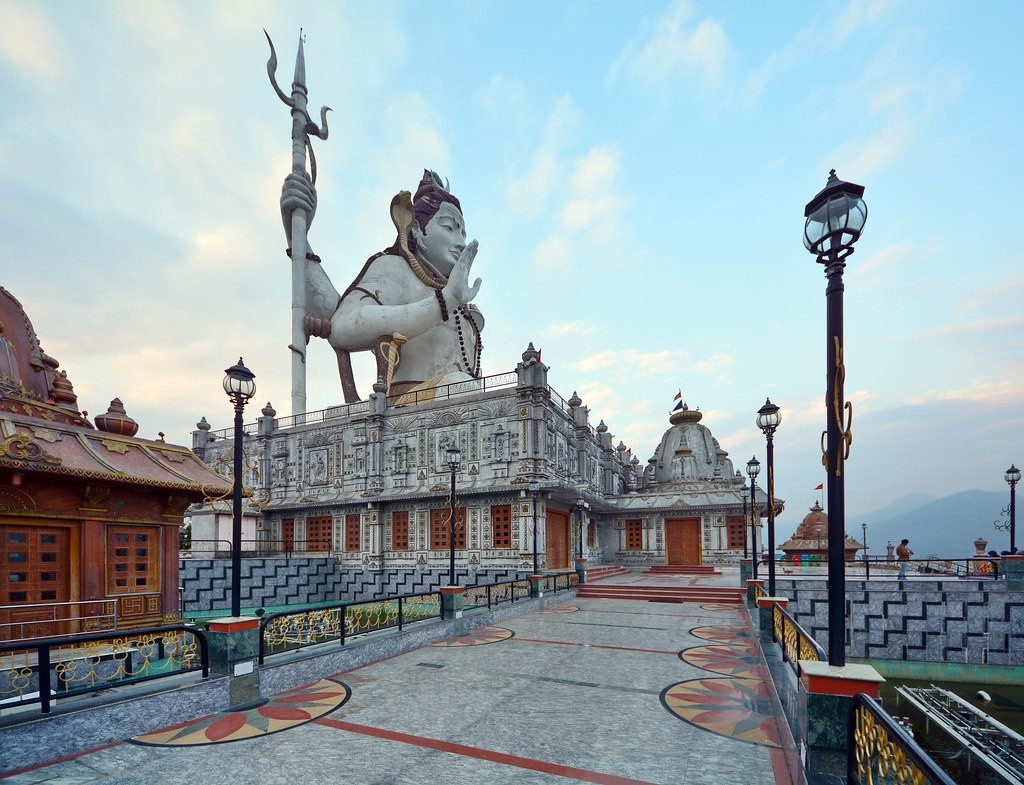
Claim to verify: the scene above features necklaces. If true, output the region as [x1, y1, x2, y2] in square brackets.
[453, 306, 482, 378]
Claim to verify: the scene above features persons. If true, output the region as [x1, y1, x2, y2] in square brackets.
[280, 168, 485, 403]
[988, 548, 1024, 556]
[896, 539, 911, 580]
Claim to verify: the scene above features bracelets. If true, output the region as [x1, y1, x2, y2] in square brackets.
[434, 288, 450, 321]
[286, 248, 322, 262]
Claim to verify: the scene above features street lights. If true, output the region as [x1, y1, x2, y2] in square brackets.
[443, 440, 462, 586]
[755, 397, 783, 596]
[528, 474, 541, 577]
[739, 483, 751, 559]
[802, 166, 870, 667]
[861, 522, 868, 554]
[221, 355, 256, 621]
[574, 493, 586, 560]
[813, 521, 823, 553]
[1004, 463, 1022, 554]
[746, 455, 762, 580]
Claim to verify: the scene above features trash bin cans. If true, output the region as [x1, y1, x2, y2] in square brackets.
[810, 554, 817, 566]
[801, 554, 809, 566]
[792, 554, 800, 566]
